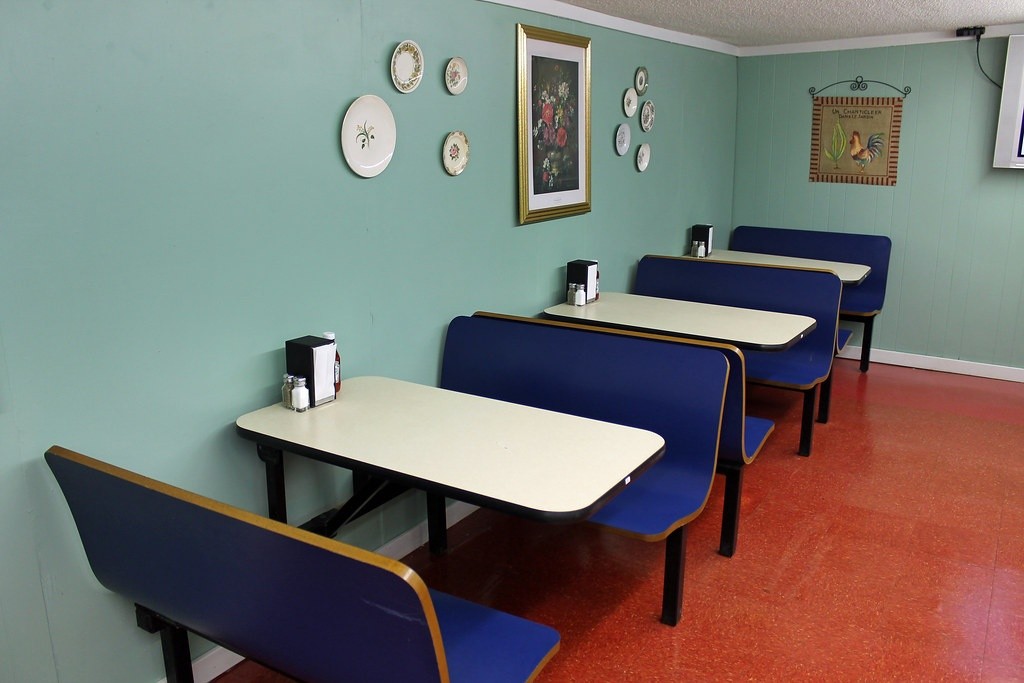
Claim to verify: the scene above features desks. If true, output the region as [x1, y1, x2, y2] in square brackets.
[236, 375, 665, 554]
[683, 249, 872, 286]
[544, 291, 817, 352]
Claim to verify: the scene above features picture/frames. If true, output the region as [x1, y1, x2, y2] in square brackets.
[516, 22, 591, 224]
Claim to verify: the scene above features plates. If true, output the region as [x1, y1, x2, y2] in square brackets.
[390, 40, 425, 94]
[443, 131, 470, 176]
[635, 66, 649, 96]
[637, 143, 650, 171]
[641, 100, 656, 132]
[445, 57, 468, 95]
[616, 124, 631, 156]
[341, 94, 397, 177]
[624, 87, 638, 118]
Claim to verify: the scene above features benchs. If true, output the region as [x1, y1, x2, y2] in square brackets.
[44, 226, 892, 683]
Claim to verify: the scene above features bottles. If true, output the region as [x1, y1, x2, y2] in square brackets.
[282, 374, 294, 408]
[291, 377, 310, 412]
[698, 241, 705, 258]
[575, 283, 586, 306]
[691, 240, 698, 258]
[567, 283, 576, 306]
[591, 259, 600, 300]
[322, 332, 341, 392]
